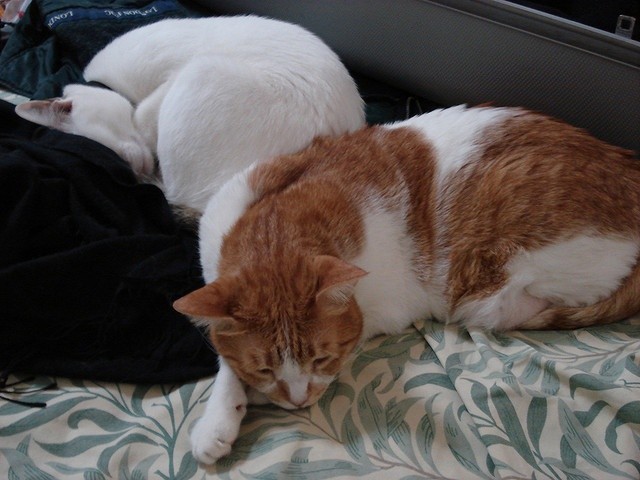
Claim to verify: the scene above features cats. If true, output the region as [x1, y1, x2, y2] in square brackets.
[172, 99, 640, 466]
[14, 13, 370, 216]
